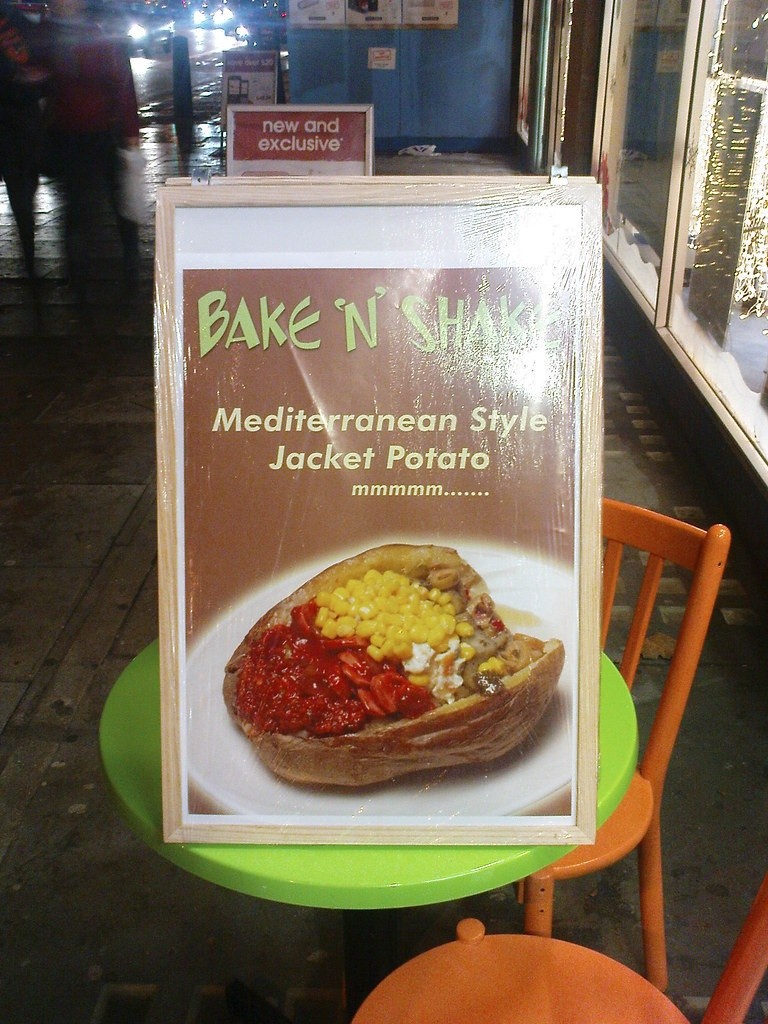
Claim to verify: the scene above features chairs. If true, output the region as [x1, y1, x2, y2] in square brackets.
[351, 873, 768, 1024]
[514, 495, 732, 995]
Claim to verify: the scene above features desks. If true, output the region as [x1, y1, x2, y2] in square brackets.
[98, 637, 639, 1024]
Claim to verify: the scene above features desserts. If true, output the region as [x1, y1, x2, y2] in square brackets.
[223, 541, 564, 786]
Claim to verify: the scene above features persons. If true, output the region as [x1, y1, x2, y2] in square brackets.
[0, 0, 144, 291]
